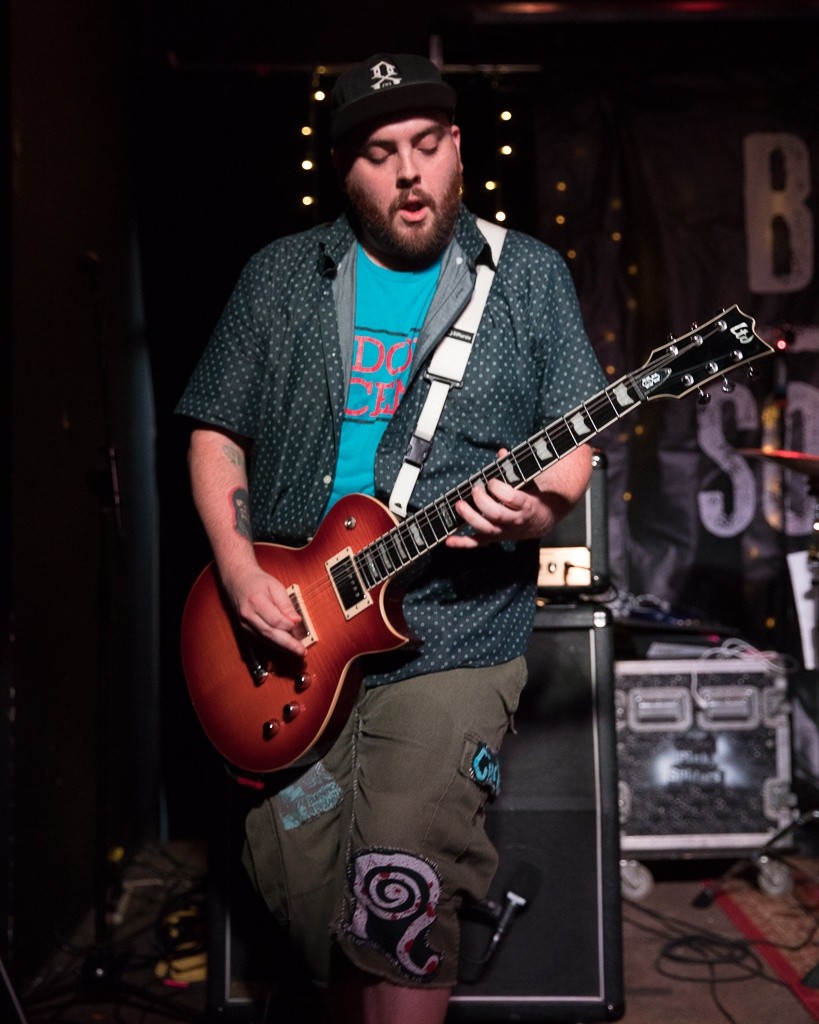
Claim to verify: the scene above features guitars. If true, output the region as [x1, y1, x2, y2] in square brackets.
[177, 302, 778, 781]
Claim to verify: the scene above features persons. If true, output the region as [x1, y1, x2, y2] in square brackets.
[174, 56, 617, 1024]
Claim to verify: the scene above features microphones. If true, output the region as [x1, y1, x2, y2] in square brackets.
[478, 877, 533, 968]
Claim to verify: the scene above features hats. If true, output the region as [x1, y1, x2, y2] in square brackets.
[333, 55, 454, 136]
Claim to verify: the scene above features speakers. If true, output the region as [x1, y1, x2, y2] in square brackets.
[207, 602, 628, 1020]
